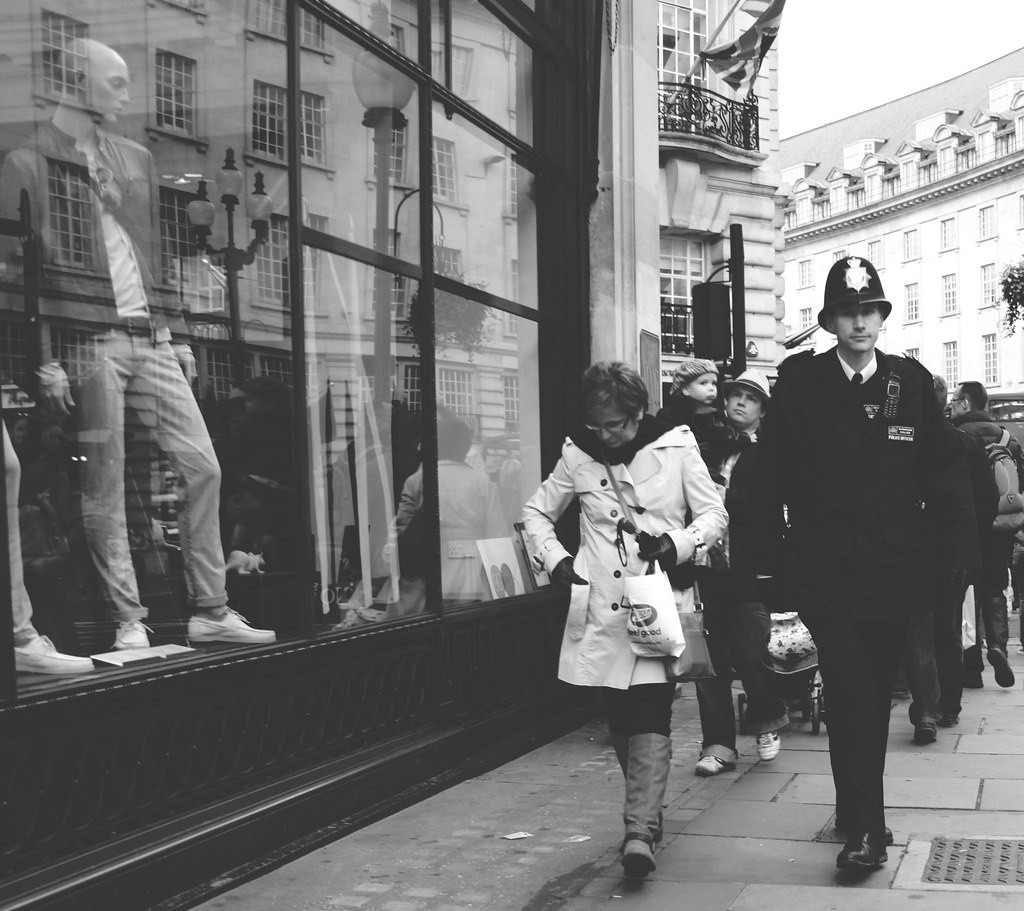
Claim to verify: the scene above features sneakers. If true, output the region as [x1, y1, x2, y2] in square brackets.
[14, 635, 95, 674]
[187, 608, 275, 645]
[756, 729, 781, 761]
[111, 618, 154, 649]
[696, 756, 727, 774]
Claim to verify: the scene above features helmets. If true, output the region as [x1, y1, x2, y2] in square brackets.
[818, 256, 892, 334]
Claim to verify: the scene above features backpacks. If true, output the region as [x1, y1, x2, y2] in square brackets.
[984, 428, 1024, 532]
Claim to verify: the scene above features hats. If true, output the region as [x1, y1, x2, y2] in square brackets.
[722, 369, 770, 406]
[669, 359, 718, 396]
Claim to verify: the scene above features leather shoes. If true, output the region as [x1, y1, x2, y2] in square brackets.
[836, 831, 887, 867]
[885, 826, 894, 843]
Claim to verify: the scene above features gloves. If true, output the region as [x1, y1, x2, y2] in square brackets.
[637, 533, 677, 571]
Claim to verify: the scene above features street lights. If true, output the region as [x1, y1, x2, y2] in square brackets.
[355, 0, 419, 591]
[189, 150, 275, 342]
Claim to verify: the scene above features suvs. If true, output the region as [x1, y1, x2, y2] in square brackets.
[484, 433, 521, 486]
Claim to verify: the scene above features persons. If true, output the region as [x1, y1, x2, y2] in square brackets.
[0, 417, 99, 676]
[519, 359, 729, 878]
[0, 38, 277, 653]
[656, 359, 1024, 777]
[729, 254, 977, 869]
[197, 373, 535, 628]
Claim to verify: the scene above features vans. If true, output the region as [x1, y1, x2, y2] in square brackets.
[944, 393, 1024, 450]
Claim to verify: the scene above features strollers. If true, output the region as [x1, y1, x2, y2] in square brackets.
[738, 610, 825, 736]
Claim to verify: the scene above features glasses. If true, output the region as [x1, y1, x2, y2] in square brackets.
[951, 399, 970, 405]
[584, 409, 634, 431]
[94, 167, 119, 209]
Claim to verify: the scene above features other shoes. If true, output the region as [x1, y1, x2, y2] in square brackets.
[621, 839, 655, 877]
[939, 715, 960, 726]
[915, 722, 936, 742]
[987, 648, 1014, 687]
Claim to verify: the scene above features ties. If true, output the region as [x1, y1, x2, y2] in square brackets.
[852, 373, 863, 385]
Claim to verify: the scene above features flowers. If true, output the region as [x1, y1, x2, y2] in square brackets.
[996, 256, 1024, 338]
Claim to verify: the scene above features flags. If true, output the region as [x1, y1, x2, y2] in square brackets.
[699, 0, 785, 100]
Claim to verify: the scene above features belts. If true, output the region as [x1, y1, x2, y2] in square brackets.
[113, 317, 154, 336]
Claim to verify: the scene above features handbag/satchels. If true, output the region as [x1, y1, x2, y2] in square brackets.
[664, 611, 716, 680]
[552, 556, 589, 592]
[625, 558, 686, 658]
[767, 612, 816, 660]
